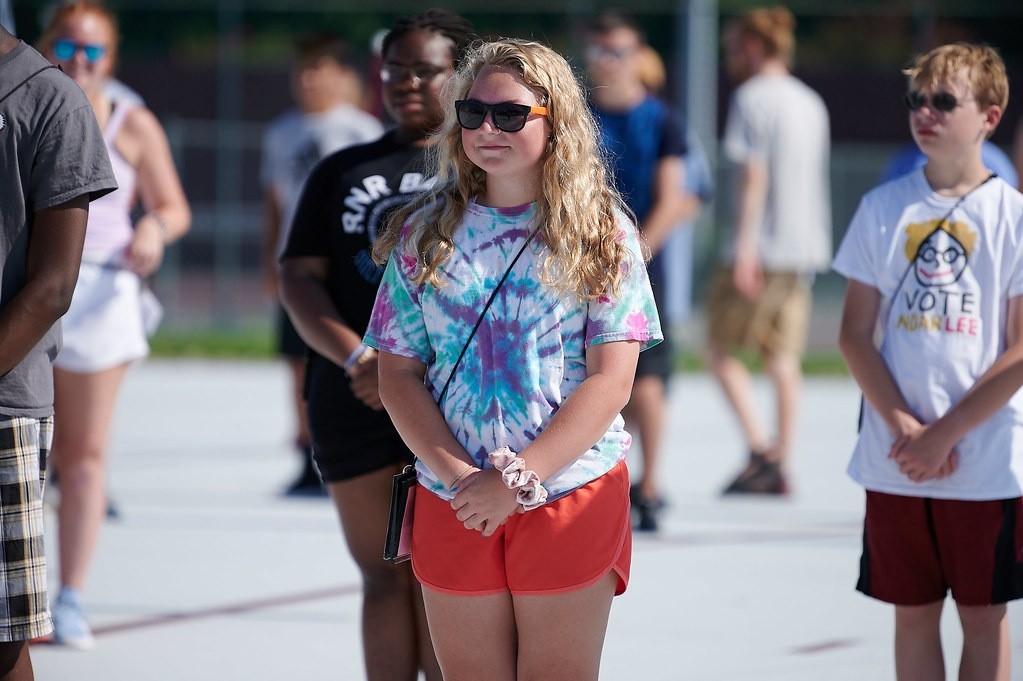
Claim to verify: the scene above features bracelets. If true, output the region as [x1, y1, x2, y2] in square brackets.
[490, 445, 548, 511]
[448, 465, 476, 489]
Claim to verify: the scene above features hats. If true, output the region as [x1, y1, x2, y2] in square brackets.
[636, 47, 666, 85]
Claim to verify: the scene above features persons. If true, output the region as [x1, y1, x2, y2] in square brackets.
[580, 16, 712, 530]
[0, 0, 191, 681]
[708, 9, 831, 493]
[343, 344, 373, 376]
[361, 39, 665, 681]
[262, 9, 476, 681]
[833, 47, 1023, 681]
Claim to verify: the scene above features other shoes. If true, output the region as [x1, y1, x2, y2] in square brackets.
[725, 452, 788, 495]
[49, 587, 94, 645]
[630, 483, 663, 535]
[283, 459, 325, 496]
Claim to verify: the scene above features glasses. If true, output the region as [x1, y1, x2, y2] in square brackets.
[454, 100, 548, 133]
[904, 89, 979, 112]
[51, 38, 109, 64]
